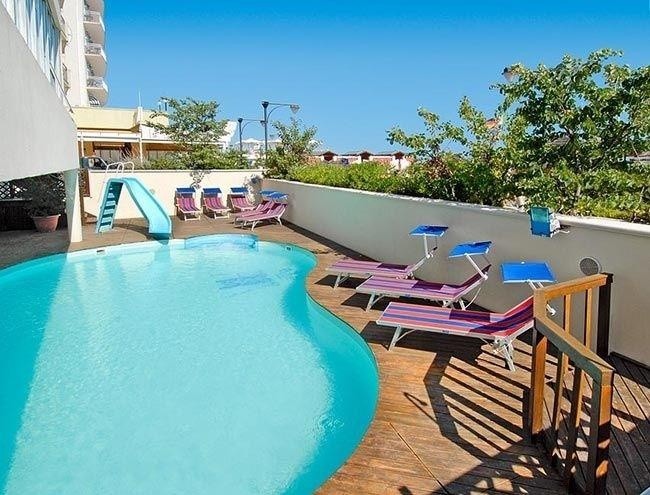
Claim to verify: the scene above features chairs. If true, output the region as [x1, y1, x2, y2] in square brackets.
[325, 223, 557, 375]
[176, 187, 288, 232]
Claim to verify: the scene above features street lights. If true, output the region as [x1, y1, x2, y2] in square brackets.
[238, 117, 265, 159]
[262, 101, 301, 161]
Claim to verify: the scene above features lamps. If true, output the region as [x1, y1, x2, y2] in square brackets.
[525, 205, 570, 239]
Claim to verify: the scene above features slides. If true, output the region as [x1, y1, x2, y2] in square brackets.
[123, 178, 172, 238]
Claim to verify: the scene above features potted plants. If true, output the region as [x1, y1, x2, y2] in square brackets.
[20, 172, 66, 234]
